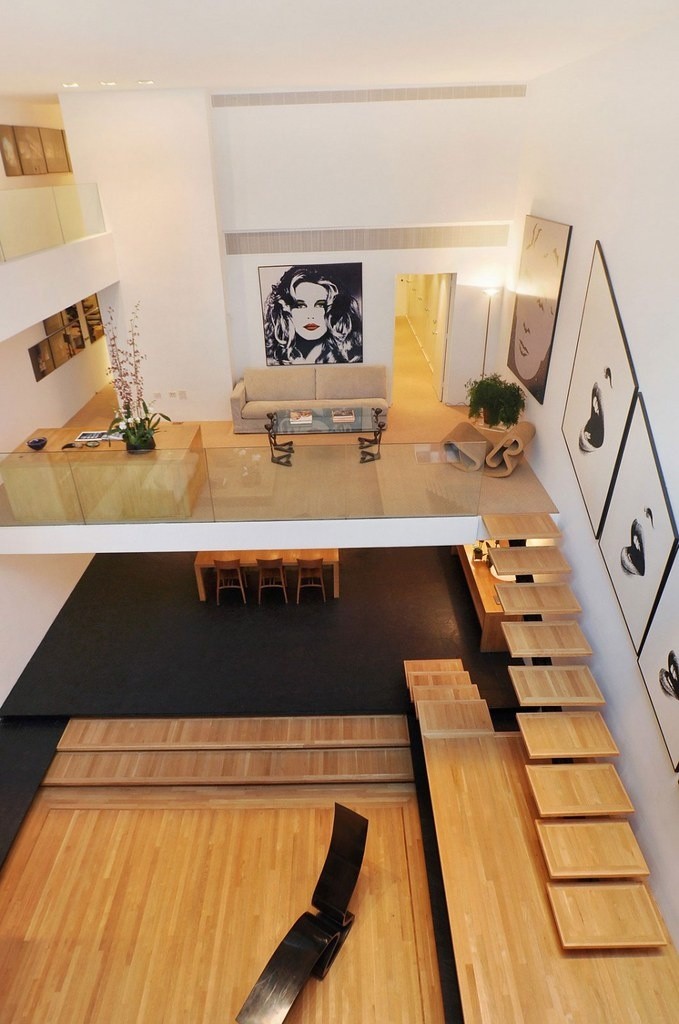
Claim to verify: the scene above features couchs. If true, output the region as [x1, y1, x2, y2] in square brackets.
[230, 365, 393, 430]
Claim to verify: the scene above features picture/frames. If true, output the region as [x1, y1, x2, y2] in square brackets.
[597, 392, 679, 656]
[560, 241, 640, 539]
[258, 261, 365, 367]
[636, 542, 679, 772]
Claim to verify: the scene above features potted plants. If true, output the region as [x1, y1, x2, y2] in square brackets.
[464, 372, 526, 429]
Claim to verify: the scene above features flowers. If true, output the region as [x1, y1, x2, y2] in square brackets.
[98, 298, 172, 446]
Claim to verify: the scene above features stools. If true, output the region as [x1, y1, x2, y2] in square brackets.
[296, 558, 326, 604]
[256, 558, 289, 605]
[213, 559, 249, 606]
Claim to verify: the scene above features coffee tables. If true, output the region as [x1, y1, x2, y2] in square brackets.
[263, 406, 388, 466]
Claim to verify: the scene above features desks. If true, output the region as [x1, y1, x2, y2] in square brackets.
[0, 426, 206, 525]
[194, 548, 340, 601]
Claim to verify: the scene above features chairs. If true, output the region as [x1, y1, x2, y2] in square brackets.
[440, 421, 536, 478]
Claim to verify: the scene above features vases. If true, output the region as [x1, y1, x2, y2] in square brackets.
[127, 434, 155, 454]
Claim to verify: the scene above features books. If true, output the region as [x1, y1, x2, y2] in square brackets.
[332, 409, 355, 423]
[289, 409, 313, 423]
